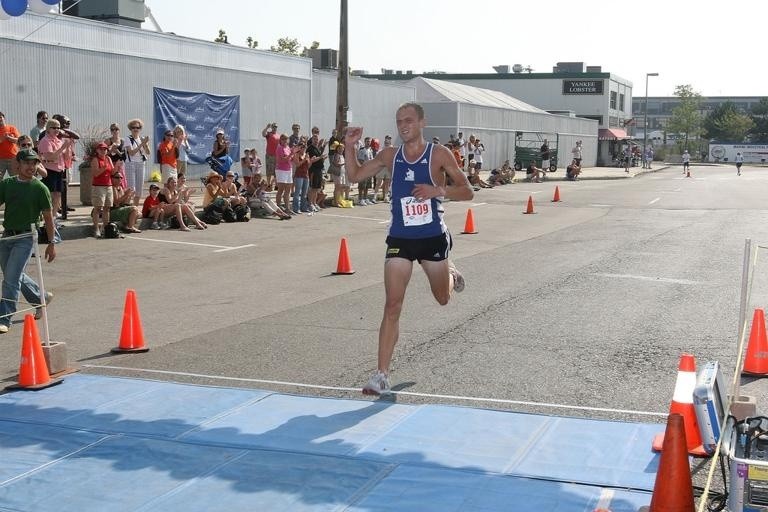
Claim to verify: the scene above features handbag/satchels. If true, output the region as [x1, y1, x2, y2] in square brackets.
[250, 199, 261, 209]
[328, 163, 341, 176]
[104, 222, 120, 239]
[203, 206, 251, 224]
[171, 217, 188, 228]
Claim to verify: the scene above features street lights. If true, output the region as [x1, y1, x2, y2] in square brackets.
[642, 72, 659, 168]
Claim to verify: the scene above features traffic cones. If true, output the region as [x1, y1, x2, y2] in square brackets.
[740, 309, 768, 379]
[460, 208, 479, 234]
[649, 355, 715, 458]
[6, 313, 63, 390]
[522, 195, 539, 215]
[551, 185, 563, 202]
[332, 237, 354, 276]
[110, 288, 149, 354]
[643, 411, 699, 511]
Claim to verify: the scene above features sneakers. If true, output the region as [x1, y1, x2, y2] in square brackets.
[127, 227, 140, 233]
[151, 222, 160, 230]
[94, 229, 100, 237]
[358, 198, 377, 206]
[362, 372, 393, 396]
[180, 222, 207, 231]
[159, 222, 170, 230]
[449, 258, 464, 293]
[0, 324, 13, 333]
[279, 203, 320, 219]
[36, 292, 53, 319]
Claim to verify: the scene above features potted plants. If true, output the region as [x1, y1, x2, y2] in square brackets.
[72, 122, 113, 206]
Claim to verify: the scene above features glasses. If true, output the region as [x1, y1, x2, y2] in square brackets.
[51, 127, 59, 129]
[131, 126, 140, 129]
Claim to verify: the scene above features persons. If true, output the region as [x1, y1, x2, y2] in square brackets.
[526, 160, 546, 183]
[612, 143, 654, 172]
[262, 122, 394, 215]
[540, 138, 552, 179]
[488, 160, 516, 186]
[203, 127, 291, 223]
[733, 152, 744, 176]
[566, 139, 585, 181]
[681, 150, 691, 174]
[433, 132, 496, 188]
[91, 119, 208, 237]
[344, 102, 474, 398]
[1, 110, 79, 331]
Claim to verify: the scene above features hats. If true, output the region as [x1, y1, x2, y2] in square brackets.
[207, 171, 223, 181]
[17, 150, 42, 163]
[98, 143, 108, 148]
